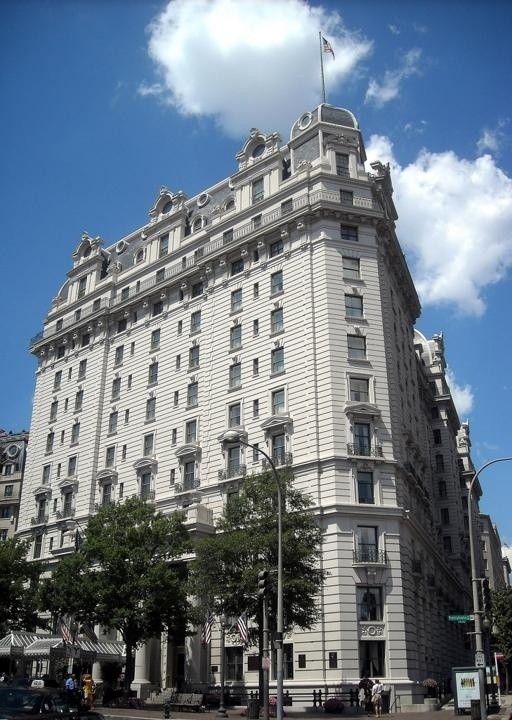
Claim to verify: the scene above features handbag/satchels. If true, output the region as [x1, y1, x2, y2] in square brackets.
[371, 695, 375, 702]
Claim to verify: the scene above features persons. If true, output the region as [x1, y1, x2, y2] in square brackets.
[370, 679, 383, 718]
[359, 672, 374, 717]
[66, 674, 78, 690]
[82, 674, 94, 711]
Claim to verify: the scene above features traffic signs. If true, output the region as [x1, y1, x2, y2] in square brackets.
[448, 615, 470, 621]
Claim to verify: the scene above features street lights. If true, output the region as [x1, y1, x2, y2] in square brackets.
[483, 617, 500, 713]
[217, 613, 228, 717]
[222, 431, 284, 719]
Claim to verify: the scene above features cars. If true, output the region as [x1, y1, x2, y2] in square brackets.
[0, 680, 90, 720]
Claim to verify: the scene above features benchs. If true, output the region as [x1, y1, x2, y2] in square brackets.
[170, 693, 205, 712]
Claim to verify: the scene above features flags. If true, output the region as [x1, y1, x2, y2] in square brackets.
[81, 621, 99, 644]
[236, 614, 249, 645]
[200, 609, 214, 645]
[60, 619, 72, 641]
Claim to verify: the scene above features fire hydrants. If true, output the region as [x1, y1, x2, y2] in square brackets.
[163, 704, 170, 718]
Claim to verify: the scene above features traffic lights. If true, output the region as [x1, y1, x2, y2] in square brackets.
[258, 569, 269, 599]
[481, 579, 491, 611]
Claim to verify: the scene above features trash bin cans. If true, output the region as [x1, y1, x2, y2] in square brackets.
[247, 699, 260, 719]
[471, 700, 481, 720]
